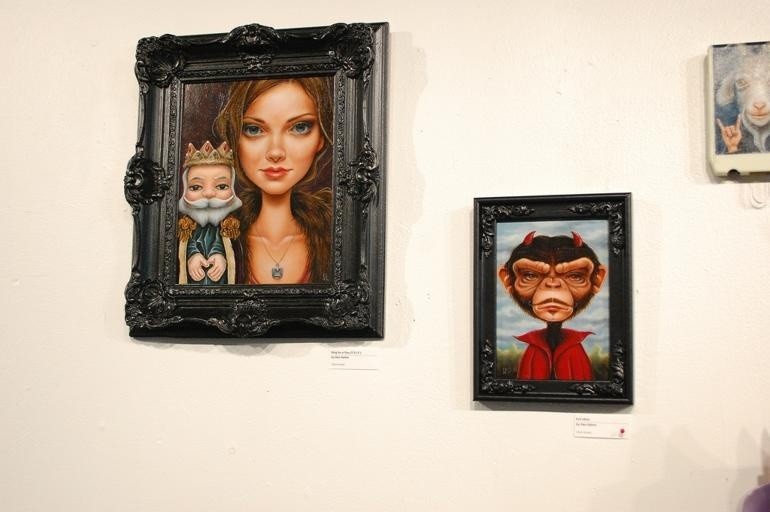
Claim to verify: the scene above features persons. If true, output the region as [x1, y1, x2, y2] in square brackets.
[179, 141, 243, 284]
[211, 74, 334, 287]
[715, 114, 743, 154]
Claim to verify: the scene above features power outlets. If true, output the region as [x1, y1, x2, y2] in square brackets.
[467, 188, 635, 410]
[118, 21, 391, 345]
[704, 39, 769, 180]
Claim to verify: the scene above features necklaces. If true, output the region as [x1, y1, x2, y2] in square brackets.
[255, 217, 298, 279]
